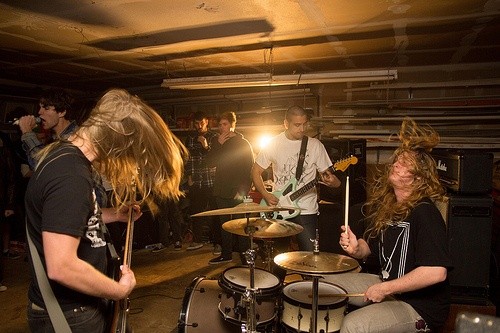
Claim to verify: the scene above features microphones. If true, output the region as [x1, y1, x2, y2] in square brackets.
[12, 117, 42, 125]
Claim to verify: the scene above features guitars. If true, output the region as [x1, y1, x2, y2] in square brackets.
[259, 155, 358, 220]
[112, 165, 138, 333]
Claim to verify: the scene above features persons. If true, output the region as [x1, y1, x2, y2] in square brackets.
[23, 88, 188, 333]
[150, 111, 255, 264]
[320, 119, 457, 333]
[250, 106, 341, 250]
[19, 86, 79, 168]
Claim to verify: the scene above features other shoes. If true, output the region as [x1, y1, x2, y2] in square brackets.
[0, 281, 8, 291]
[4, 250, 21, 259]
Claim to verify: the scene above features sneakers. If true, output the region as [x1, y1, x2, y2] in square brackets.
[209, 255, 232, 264]
[151, 242, 167, 253]
[214, 243, 223, 255]
[174, 240, 184, 250]
[187, 242, 203, 250]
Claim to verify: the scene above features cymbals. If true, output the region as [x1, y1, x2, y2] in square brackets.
[191, 207, 306, 219]
[273, 252, 359, 273]
[223, 219, 303, 237]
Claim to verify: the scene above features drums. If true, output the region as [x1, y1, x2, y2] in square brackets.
[281, 280, 349, 333]
[177, 274, 241, 333]
[218, 266, 281, 326]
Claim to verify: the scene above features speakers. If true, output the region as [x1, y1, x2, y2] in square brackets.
[426, 149, 496, 289]
[319, 138, 369, 257]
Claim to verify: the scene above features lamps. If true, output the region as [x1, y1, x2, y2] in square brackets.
[272, 44, 401, 87]
[160, 47, 274, 89]
